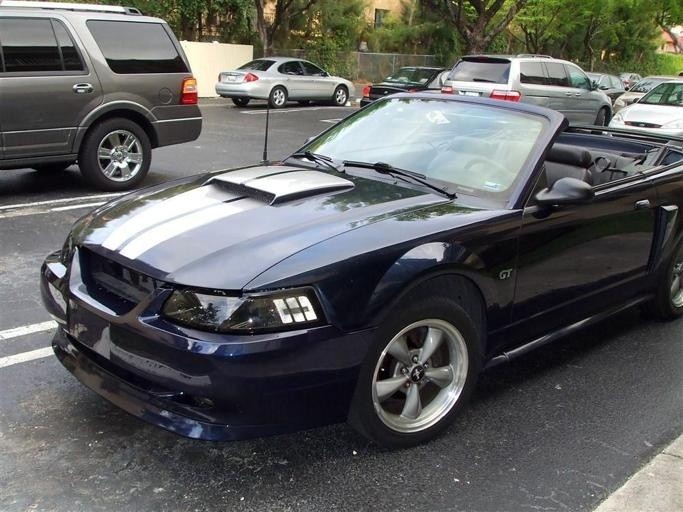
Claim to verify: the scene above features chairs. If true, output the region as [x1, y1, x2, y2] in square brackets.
[426, 131, 638, 198]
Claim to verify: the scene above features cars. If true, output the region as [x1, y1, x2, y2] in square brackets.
[215, 57, 356, 108]
[0, 0, 203, 191]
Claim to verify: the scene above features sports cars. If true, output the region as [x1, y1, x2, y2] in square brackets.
[39, 91, 683, 449]
